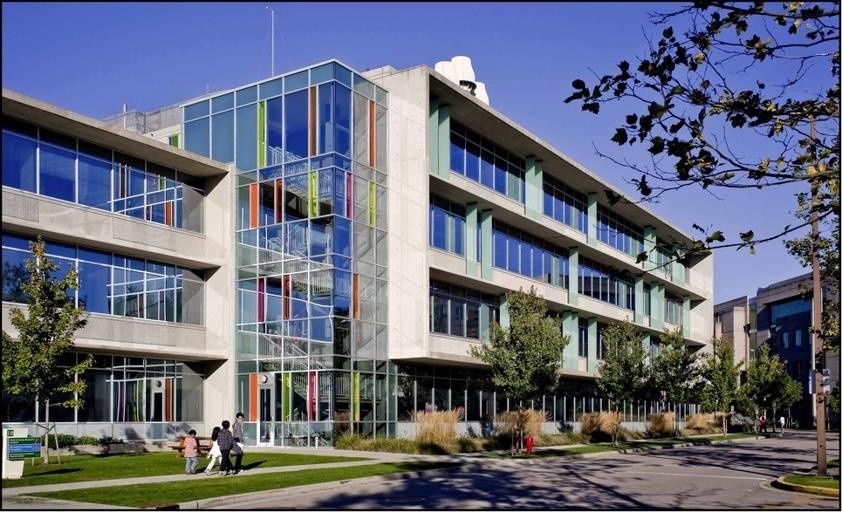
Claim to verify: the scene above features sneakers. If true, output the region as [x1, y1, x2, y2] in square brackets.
[185, 468, 196, 473]
[205, 469, 244, 476]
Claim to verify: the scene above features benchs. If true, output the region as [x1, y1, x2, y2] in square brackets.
[168, 444, 212, 458]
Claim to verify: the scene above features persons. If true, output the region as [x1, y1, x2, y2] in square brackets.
[216, 420, 234, 476]
[231, 413, 246, 472]
[525, 433, 536, 456]
[182, 429, 200, 474]
[203, 427, 223, 476]
[750, 414, 768, 434]
[778, 415, 786, 432]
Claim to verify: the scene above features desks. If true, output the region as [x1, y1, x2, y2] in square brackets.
[176, 435, 212, 458]
